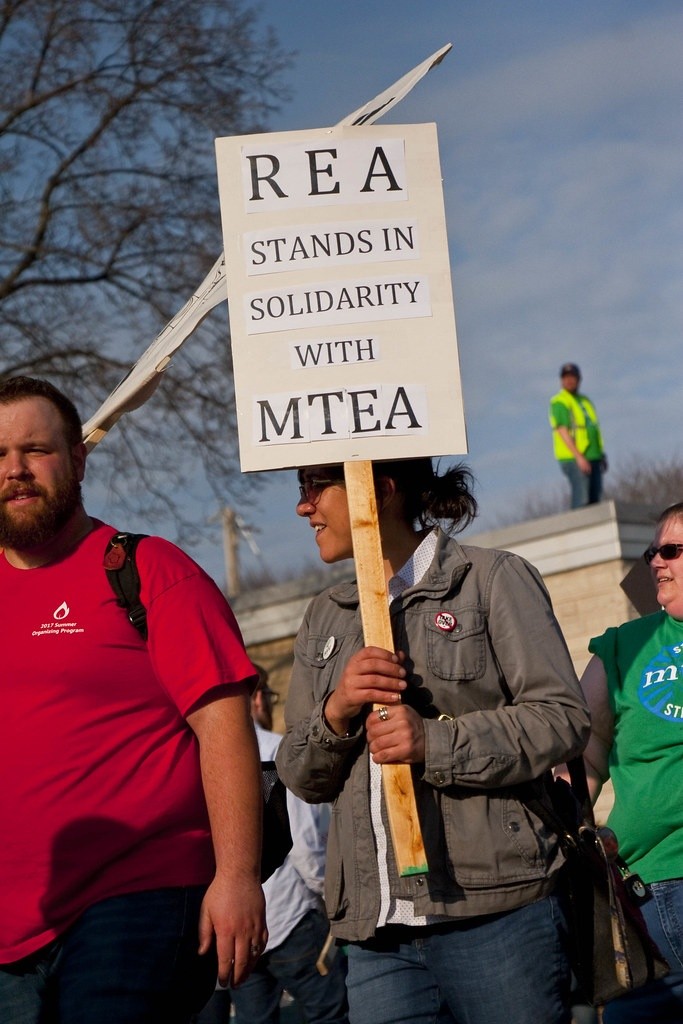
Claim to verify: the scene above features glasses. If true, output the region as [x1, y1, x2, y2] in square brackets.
[299, 480, 346, 502]
[265, 691, 280, 705]
[644, 544, 683, 566]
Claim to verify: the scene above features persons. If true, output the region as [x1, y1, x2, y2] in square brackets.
[276, 456, 591, 1024]
[230, 662, 348, 1024]
[553, 504, 682, 1024]
[549, 363, 608, 510]
[0, 375, 270, 1024]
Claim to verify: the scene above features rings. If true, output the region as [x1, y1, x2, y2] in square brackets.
[379, 707, 389, 721]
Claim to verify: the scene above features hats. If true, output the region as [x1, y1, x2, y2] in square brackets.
[561, 363, 578, 378]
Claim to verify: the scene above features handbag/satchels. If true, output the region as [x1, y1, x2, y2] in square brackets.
[551, 837, 671, 1010]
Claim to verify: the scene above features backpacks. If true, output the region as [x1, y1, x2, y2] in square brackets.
[104, 532, 293, 885]
